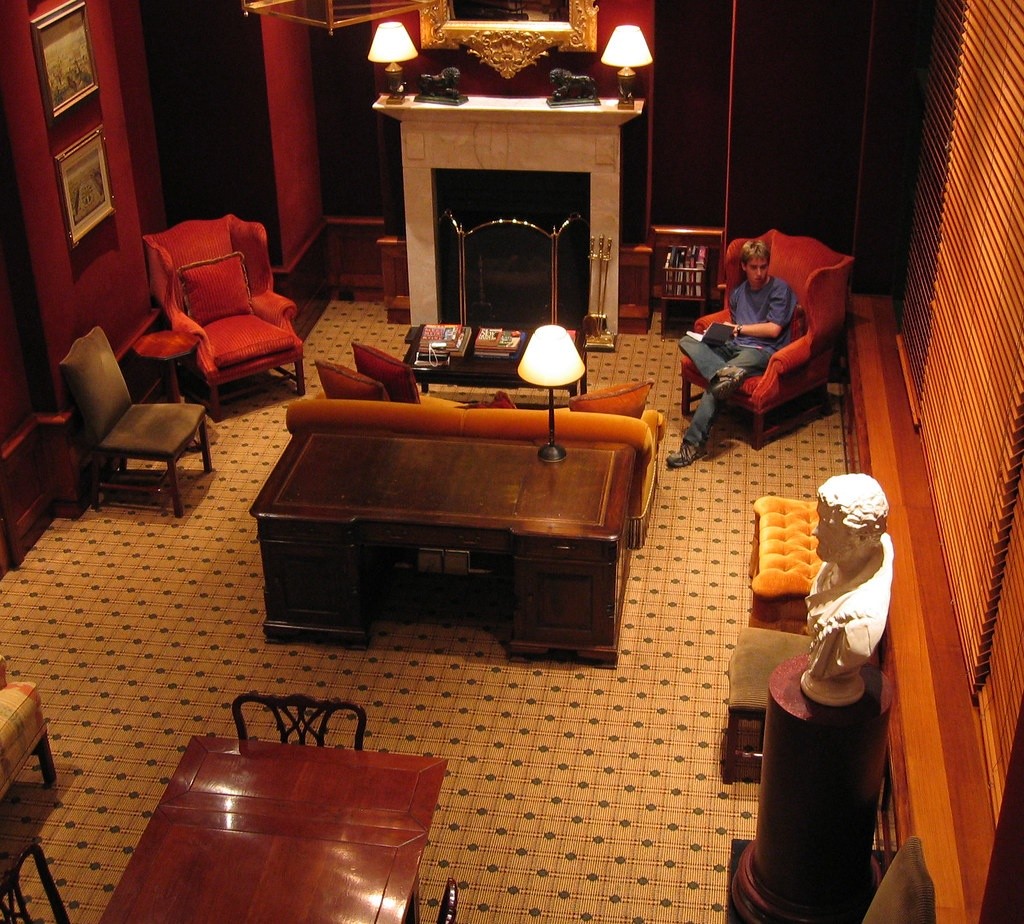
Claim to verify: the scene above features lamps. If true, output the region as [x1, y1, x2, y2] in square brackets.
[602, 25, 653, 110]
[516, 325, 586, 464]
[368, 21, 419, 104]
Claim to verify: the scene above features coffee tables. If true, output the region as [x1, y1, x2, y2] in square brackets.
[404, 323, 588, 407]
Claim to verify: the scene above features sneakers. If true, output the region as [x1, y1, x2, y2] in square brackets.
[712, 367, 748, 400]
[667, 442, 708, 467]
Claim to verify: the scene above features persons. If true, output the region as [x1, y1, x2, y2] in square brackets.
[667, 240, 796, 467]
[807, 472, 894, 683]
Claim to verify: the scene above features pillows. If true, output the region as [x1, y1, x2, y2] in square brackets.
[568, 378, 657, 418]
[456, 391, 514, 410]
[314, 358, 390, 404]
[179, 251, 251, 328]
[351, 339, 420, 403]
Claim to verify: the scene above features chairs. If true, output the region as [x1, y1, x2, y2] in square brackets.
[436, 876, 458, 924]
[682, 229, 855, 453]
[60, 326, 214, 516]
[0, 837, 71, 924]
[141, 215, 306, 422]
[231, 691, 367, 752]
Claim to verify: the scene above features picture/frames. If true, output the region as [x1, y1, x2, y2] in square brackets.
[418, 1, 598, 79]
[53, 125, 116, 251]
[30, 0, 100, 131]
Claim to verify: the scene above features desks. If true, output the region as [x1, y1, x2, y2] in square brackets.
[251, 435, 636, 666]
[97, 737, 448, 924]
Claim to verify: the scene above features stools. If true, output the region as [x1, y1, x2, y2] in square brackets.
[719, 626, 812, 782]
[748, 492, 819, 636]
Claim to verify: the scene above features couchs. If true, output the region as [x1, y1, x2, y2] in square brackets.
[0, 653, 56, 803]
[286, 374, 664, 549]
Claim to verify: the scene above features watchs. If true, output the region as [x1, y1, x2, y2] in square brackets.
[737, 324, 742, 335]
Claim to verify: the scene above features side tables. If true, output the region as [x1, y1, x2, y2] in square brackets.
[134, 333, 200, 402]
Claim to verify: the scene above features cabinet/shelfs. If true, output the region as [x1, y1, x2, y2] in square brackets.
[661, 244, 707, 343]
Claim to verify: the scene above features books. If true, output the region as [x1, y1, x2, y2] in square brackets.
[473, 326, 526, 360]
[686, 321, 735, 348]
[418, 324, 472, 357]
[665, 246, 707, 296]
